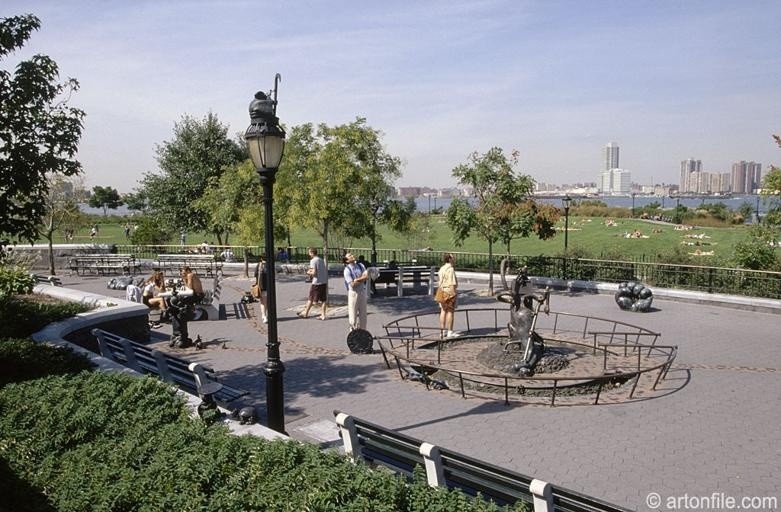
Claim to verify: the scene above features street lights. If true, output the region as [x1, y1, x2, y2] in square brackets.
[433, 194, 438, 209]
[245, 90, 286, 436]
[756, 197, 760, 216]
[631, 193, 636, 215]
[702, 196, 706, 203]
[370, 202, 378, 263]
[562, 194, 571, 248]
[662, 196, 665, 207]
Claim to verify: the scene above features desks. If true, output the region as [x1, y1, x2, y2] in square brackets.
[156, 289, 192, 299]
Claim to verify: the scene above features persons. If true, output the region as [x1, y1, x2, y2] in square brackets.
[614, 281, 654, 313]
[550, 210, 781, 256]
[358, 255, 371, 267]
[341, 252, 369, 332]
[63, 222, 291, 324]
[434, 253, 460, 338]
[296, 246, 328, 321]
[382, 259, 398, 289]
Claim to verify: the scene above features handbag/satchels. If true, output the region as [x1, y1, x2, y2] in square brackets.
[434, 288, 450, 303]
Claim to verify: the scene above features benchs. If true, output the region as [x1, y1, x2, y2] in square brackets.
[91, 327, 252, 404]
[333, 409, 634, 512]
[69, 256, 224, 278]
[376, 263, 439, 288]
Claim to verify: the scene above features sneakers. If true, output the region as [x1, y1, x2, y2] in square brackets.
[440, 331, 460, 338]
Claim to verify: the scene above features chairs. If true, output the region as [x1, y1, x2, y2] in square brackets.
[126, 285, 143, 302]
